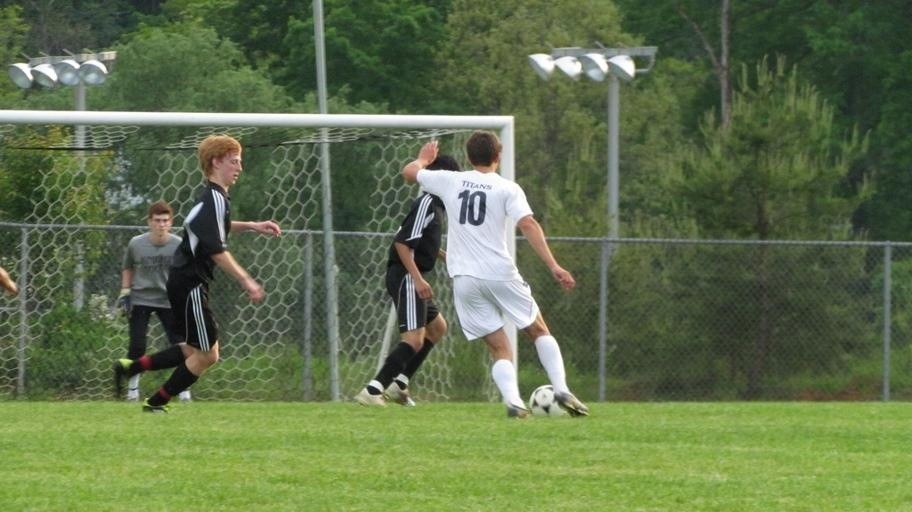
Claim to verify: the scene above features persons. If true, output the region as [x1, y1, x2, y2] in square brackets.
[115, 132, 283, 414]
[1, 267, 19, 296]
[115, 200, 192, 404]
[354, 154, 462, 409]
[402, 128, 594, 419]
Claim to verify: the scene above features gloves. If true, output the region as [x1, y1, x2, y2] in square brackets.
[112, 288, 133, 326]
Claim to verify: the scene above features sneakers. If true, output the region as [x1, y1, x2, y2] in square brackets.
[140, 398, 178, 417]
[111, 357, 132, 400]
[551, 391, 590, 418]
[347, 387, 388, 411]
[384, 381, 417, 408]
[504, 399, 533, 419]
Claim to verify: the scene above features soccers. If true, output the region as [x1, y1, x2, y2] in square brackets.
[528, 384, 568, 416]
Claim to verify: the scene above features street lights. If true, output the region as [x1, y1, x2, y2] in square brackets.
[521, 40, 666, 254]
[4, 46, 121, 312]
